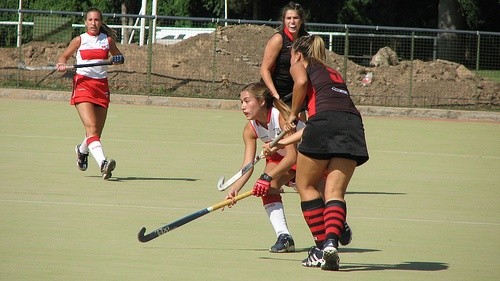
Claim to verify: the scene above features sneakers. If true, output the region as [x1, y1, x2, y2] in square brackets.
[300, 245, 324, 267]
[338, 222, 353, 246]
[320, 238, 340, 270]
[269, 233, 295, 252]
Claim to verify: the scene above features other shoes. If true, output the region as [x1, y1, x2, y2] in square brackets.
[100, 156, 116, 180]
[75, 144, 89, 171]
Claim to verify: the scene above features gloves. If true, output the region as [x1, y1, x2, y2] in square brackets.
[252, 173, 272, 197]
[110, 54, 124, 64]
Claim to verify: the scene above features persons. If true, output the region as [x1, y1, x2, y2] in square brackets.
[263, 34, 369, 271]
[259, 1, 310, 193]
[222, 82, 352, 252]
[56, 7, 124, 180]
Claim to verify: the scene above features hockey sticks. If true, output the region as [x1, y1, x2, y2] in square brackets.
[18, 61, 112, 71]
[138, 190, 252, 243]
[216, 130, 285, 191]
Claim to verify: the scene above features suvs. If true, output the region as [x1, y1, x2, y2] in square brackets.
[146, 30, 198, 47]
